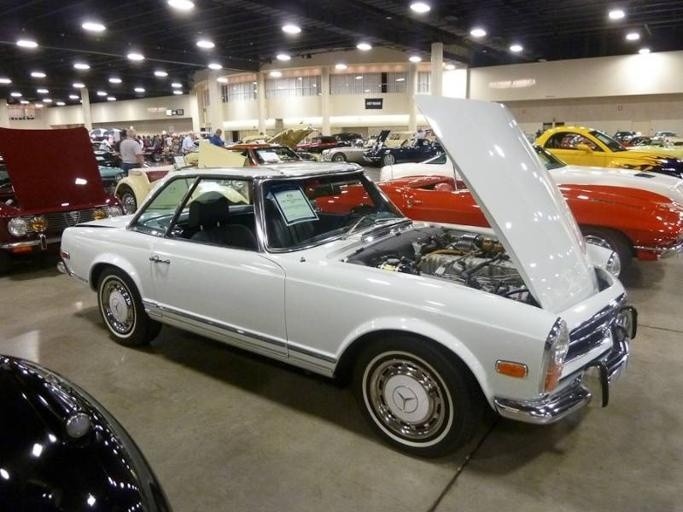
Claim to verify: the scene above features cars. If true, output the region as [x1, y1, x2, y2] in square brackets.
[302, 168, 683, 283]
[530, 124, 683, 173]
[614, 129, 683, 146]
[91, 125, 445, 215]
[378, 140, 683, 209]
[0, 354, 173, 512]
[54, 96, 640, 458]
[0, 125, 110, 272]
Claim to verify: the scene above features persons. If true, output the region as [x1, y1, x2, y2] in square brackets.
[116, 127, 196, 174]
[209, 129, 226, 148]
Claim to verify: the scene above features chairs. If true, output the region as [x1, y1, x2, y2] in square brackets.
[191, 192, 258, 253]
